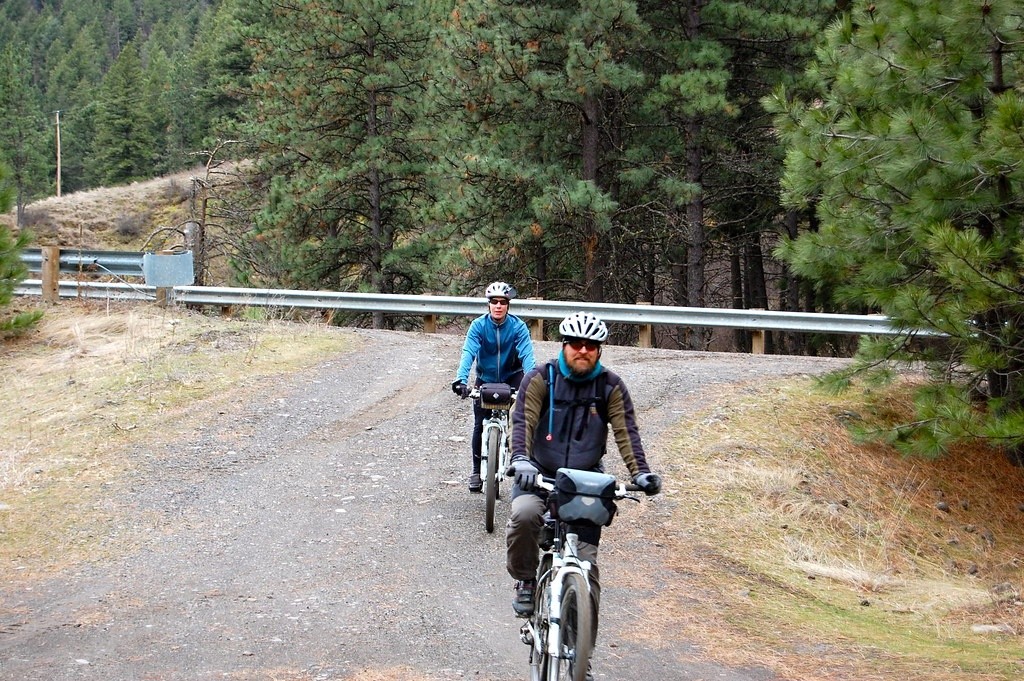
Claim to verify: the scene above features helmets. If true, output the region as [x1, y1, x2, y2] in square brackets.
[485, 281, 516, 299]
[559, 310, 608, 341]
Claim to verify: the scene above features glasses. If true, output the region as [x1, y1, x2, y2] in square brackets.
[489, 299, 508, 304]
[563, 339, 599, 350]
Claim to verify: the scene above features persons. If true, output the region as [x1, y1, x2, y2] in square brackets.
[506, 312, 662, 681]
[452, 282, 535, 492]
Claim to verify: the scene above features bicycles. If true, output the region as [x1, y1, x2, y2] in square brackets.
[506, 466, 645, 680]
[467, 383, 518, 532]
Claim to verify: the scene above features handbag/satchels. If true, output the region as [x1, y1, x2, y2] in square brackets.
[480, 382, 512, 409]
[556, 467, 615, 526]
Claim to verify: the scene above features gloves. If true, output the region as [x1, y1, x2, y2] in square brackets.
[452, 383, 468, 398]
[511, 454, 538, 490]
[632, 472, 662, 495]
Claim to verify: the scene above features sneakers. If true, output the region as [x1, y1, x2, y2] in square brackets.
[512, 579, 536, 614]
[468, 473, 483, 490]
[585, 662, 594, 681]
